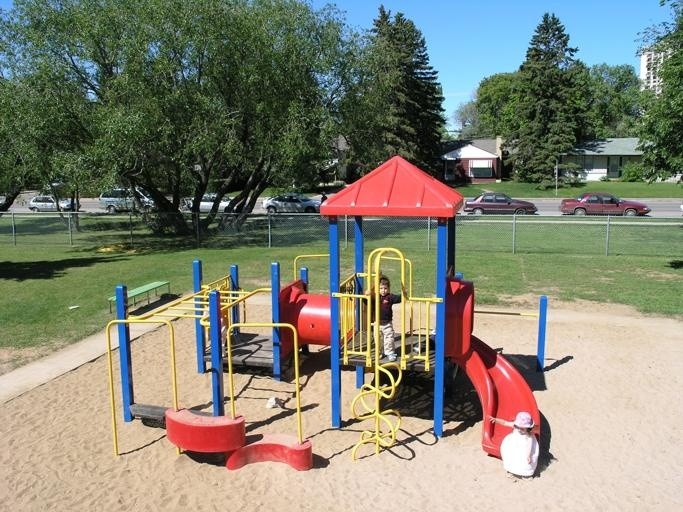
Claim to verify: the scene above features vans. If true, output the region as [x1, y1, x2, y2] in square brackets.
[99, 188, 171, 214]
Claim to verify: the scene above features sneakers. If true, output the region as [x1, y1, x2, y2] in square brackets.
[388, 354, 398, 362]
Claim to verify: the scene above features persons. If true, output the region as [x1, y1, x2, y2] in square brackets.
[320, 191, 327, 218]
[363, 277, 408, 362]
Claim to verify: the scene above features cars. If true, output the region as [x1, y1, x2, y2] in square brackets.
[185, 194, 247, 213]
[262, 194, 323, 214]
[464, 192, 539, 217]
[29, 194, 82, 213]
[559, 192, 652, 217]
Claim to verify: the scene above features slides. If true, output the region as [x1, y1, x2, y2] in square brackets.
[451, 335, 541, 459]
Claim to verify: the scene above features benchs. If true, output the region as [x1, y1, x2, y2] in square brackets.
[107, 280, 170, 314]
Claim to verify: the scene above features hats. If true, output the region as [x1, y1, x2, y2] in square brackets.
[514, 412, 534, 428]
